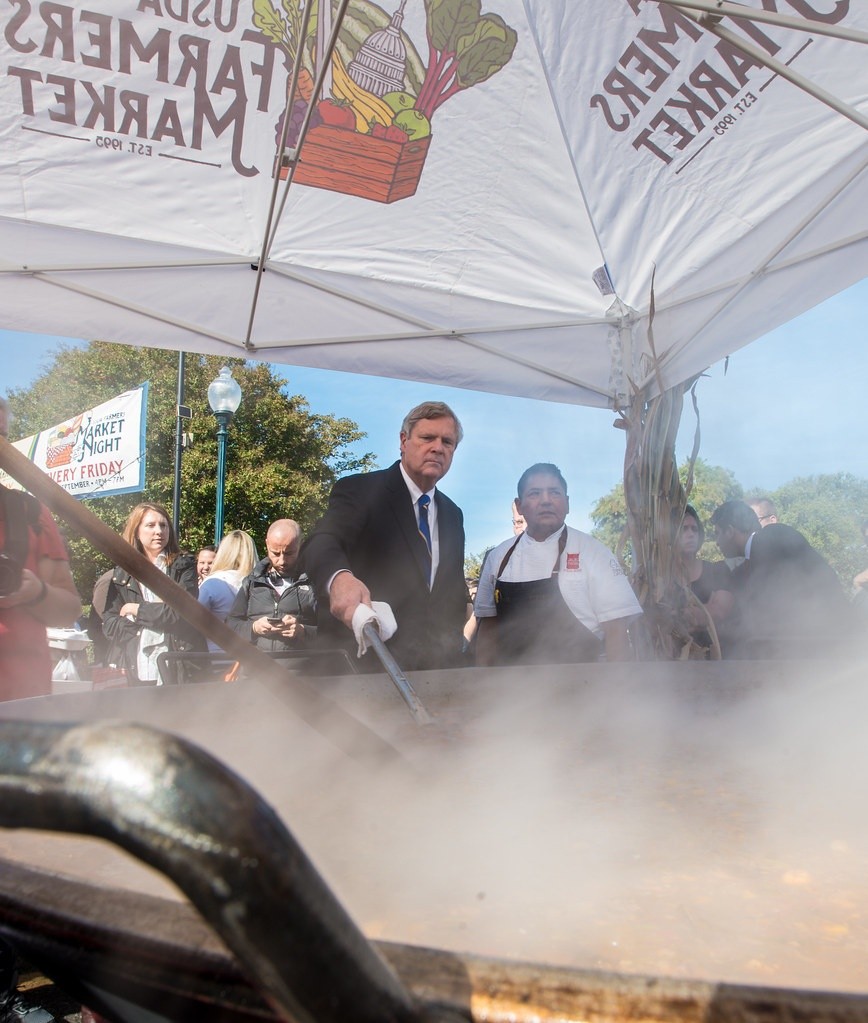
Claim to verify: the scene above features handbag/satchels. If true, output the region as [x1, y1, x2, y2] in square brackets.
[224, 660, 240, 682]
[51, 653, 81, 681]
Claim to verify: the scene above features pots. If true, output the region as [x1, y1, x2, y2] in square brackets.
[0, 652, 868, 1023]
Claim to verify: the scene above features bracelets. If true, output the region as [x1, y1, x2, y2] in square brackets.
[25, 583, 47, 607]
[254, 622, 259, 635]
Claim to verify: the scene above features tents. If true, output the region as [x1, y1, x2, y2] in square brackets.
[1, 0, 868, 662]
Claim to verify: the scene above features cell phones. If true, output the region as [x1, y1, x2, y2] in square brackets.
[267, 618, 283, 628]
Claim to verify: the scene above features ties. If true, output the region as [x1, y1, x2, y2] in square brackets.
[417, 495, 432, 591]
[744, 558, 749, 576]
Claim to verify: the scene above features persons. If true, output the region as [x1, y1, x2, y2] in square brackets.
[102, 502, 319, 687]
[711, 499, 848, 660]
[853, 518, 868, 588]
[680, 503, 741, 661]
[0, 397, 81, 702]
[464, 462, 642, 663]
[723, 498, 776, 571]
[302, 401, 473, 671]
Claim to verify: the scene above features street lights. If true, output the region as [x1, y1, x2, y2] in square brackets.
[205, 364, 242, 551]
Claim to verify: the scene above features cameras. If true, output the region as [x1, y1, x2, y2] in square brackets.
[0, 551, 23, 596]
